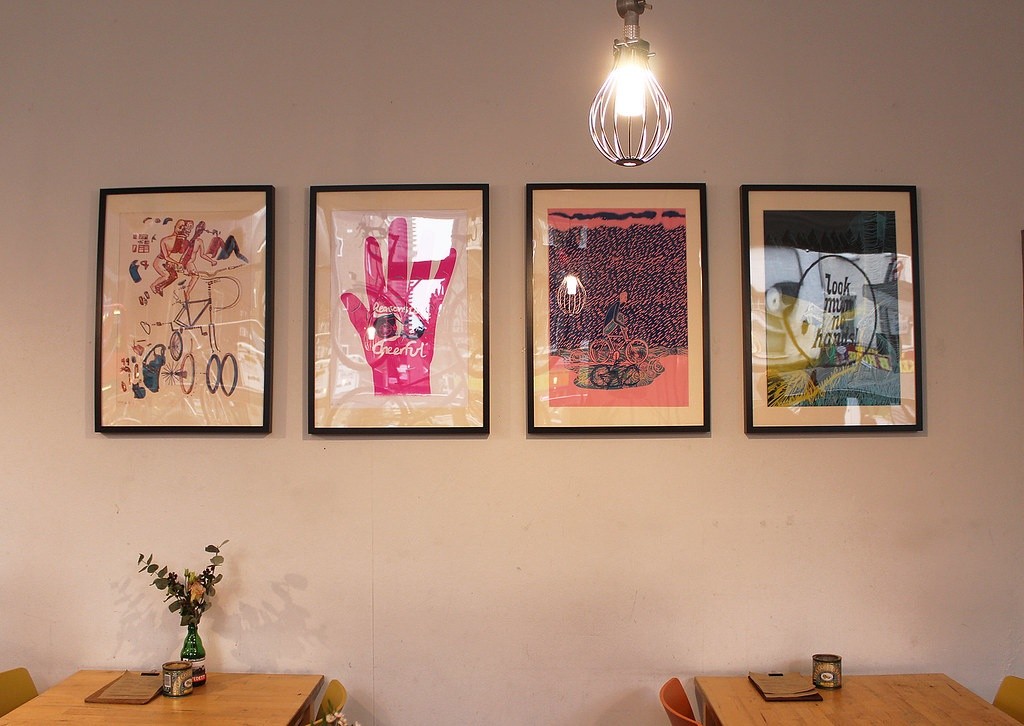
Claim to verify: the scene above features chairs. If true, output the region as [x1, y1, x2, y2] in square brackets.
[660, 677, 701, 726]
[0, 668, 38, 716]
[993, 676, 1024, 726]
[310, 680, 346, 726]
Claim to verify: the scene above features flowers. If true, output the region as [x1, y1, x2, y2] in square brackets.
[311, 699, 361, 726]
[138, 539, 228, 627]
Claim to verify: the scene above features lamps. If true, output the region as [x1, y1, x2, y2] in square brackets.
[588, 0, 671, 165]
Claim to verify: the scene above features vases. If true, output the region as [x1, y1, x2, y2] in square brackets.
[179, 623, 207, 688]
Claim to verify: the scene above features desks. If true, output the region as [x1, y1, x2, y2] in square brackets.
[694, 673, 1024, 726]
[0, 672, 323, 726]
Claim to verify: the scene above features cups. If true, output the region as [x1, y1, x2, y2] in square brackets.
[812, 654, 842, 690]
[163, 662, 193, 698]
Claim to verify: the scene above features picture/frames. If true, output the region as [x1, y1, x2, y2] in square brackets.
[305, 182, 489, 438]
[524, 182, 709, 434]
[95, 184, 275, 435]
[739, 182, 924, 432]
